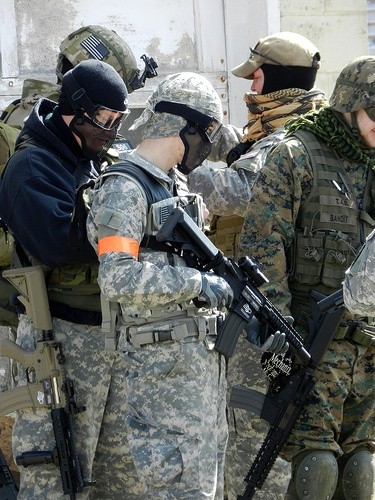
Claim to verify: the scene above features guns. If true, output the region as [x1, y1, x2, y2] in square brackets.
[228, 288, 346, 500]
[155, 208, 311, 365]
[0, 266, 96, 500]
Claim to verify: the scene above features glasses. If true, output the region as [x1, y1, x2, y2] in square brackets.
[156, 100, 225, 151]
[92, 104, 131, 131]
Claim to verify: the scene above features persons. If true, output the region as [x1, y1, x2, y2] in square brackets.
[0, 27, 327, 499]
[239, 56, 375, 500]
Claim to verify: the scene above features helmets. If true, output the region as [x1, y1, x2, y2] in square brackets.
[328, 56, 375, 111]
[54, 24, 139, 94]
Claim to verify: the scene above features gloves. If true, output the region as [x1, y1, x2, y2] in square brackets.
[200, 271, 234, 311]
[244, 311, 294, 354]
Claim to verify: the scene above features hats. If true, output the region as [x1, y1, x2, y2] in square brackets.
[57, 59, 129, 115]
[230, 31, 321, 81]
